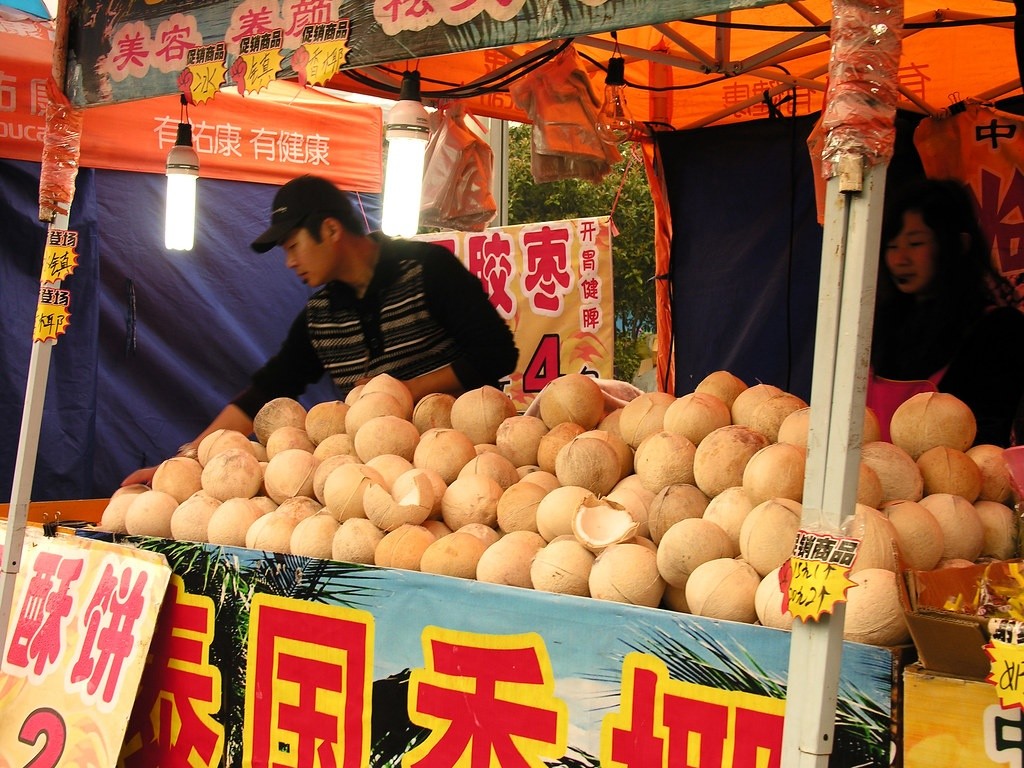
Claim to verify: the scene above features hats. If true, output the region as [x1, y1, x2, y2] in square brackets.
[251, 173, 348, 253]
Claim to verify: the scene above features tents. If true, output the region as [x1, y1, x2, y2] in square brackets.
[0, 0, 1024, 768]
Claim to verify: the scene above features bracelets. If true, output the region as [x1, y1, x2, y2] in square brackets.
[178, 444, 199, 461]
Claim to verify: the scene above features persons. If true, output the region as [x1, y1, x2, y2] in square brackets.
[120, 176, 519, 486]
[871, 177, 1024, 446]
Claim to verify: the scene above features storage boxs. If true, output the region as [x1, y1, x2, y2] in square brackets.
[888, 535, 1024, 680]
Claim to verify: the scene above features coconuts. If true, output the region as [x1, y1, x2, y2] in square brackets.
[98, 373, 1017, 649]
